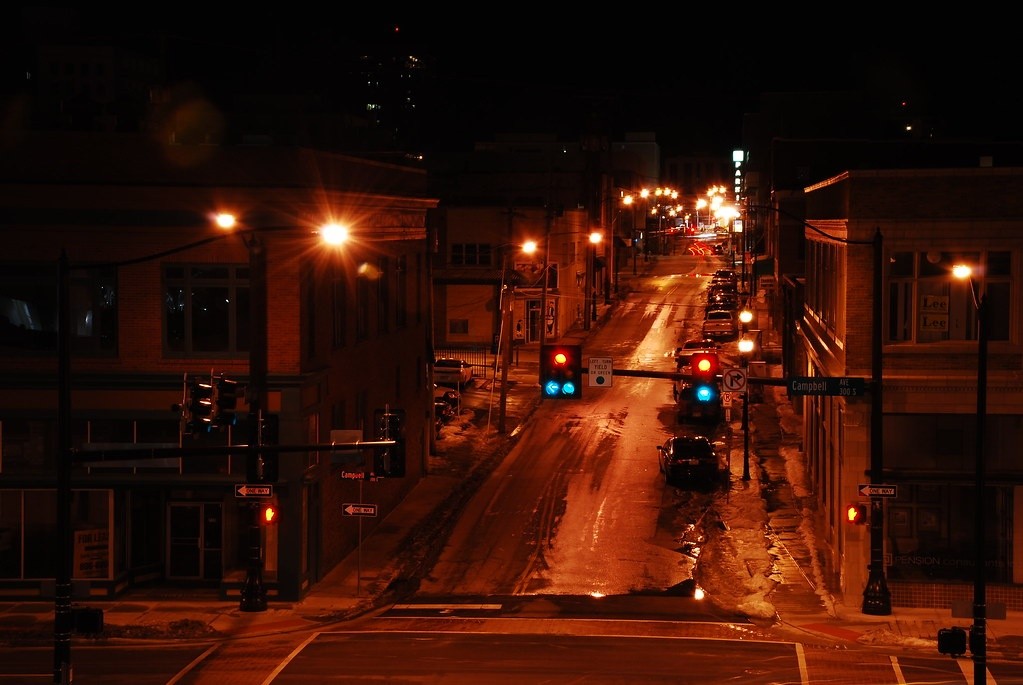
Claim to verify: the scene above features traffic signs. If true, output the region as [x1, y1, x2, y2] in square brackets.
[342, 503, 378, 518]
[234, 484, 274, 499]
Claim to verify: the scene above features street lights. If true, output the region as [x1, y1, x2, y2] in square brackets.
[607, 195, 633, 306]
[719, 204, 892, 615]
[52, 219, 353, 685]
[427, 240, 536, 455]
[947, 248, 989, 685]
[539, 220, 603, 345]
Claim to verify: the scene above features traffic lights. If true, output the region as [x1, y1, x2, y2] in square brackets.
[215, 374, 239, 427]
[691, 352, 721, 409]
[858, 484, 899, 498]
[540, 344, 583, 400]
[189, 380, 213, 430]
[846, 505, 863, 523]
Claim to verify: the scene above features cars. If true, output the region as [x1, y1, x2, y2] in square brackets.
[713, 225, 737, 254]
[433, 357, 473, 391]
[433, 383, 463, 423]
[711, 244, 724, 256]
[657, 435, 719, 486]
[674, 270, 738, 379]
[676, 382, 722, 426]
[753, 329, 782, 367]
